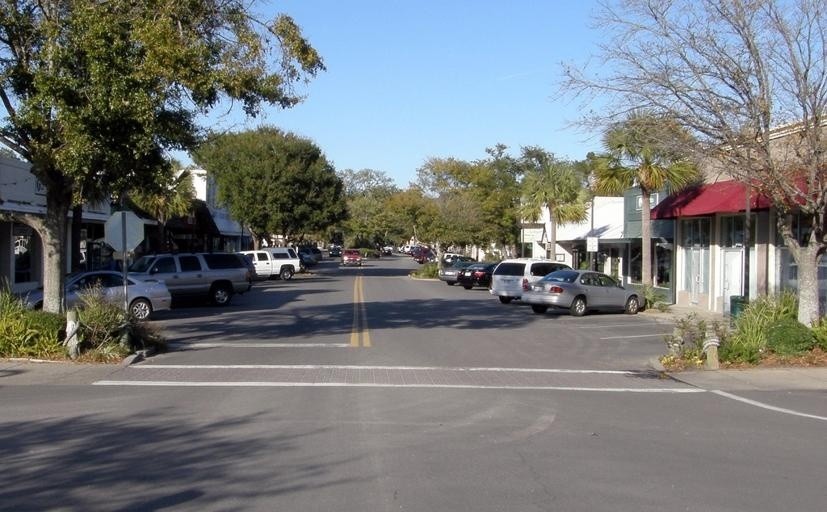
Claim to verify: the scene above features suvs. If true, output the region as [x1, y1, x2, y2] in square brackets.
[126, 252, 255, 305]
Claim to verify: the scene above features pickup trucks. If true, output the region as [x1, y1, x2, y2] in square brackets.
[236, 247, 302, 281]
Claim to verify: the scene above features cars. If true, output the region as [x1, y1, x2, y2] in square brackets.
[295, 245, 323, 265]
[381, 247, 392, 256]
[18, 270, 172, 321]
[340, 250, 363, 267]
[328, 247, 339, 257]
[398, 242, 646, 316]
